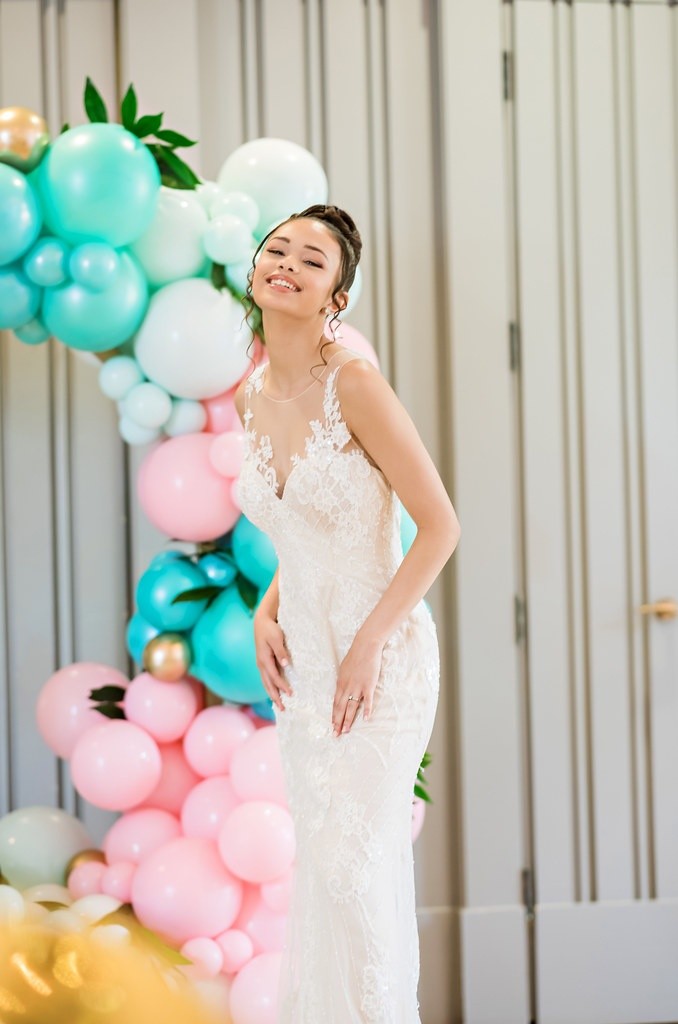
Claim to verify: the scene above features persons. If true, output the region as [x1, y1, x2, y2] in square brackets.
[228, 206, 461, 1024]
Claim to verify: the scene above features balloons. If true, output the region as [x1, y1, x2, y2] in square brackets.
[0, 108, 427, 970]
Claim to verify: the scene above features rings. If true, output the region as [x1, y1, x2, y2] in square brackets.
[348, 696, 361, 702]
